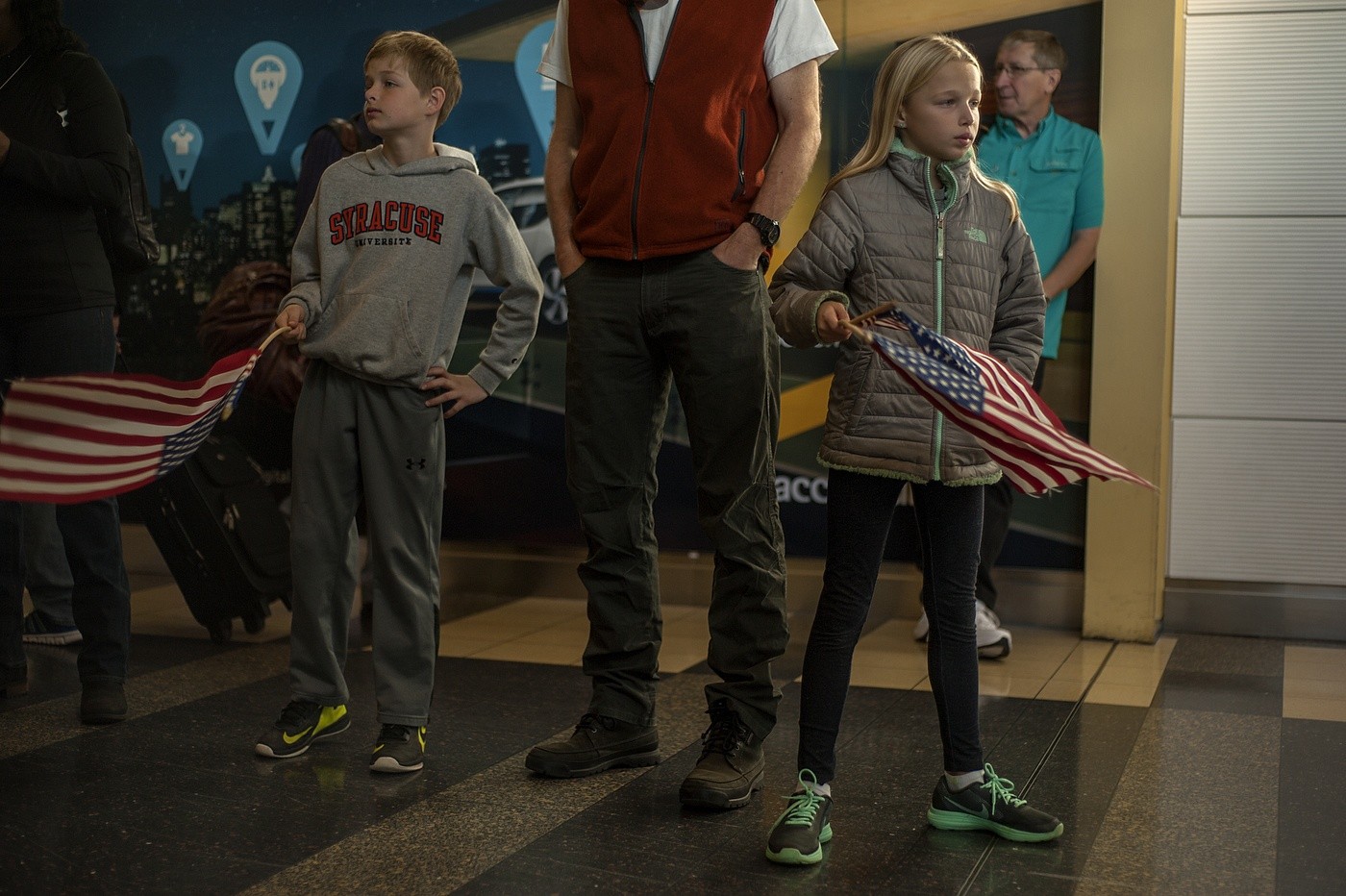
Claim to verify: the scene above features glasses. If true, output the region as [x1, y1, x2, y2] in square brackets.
[995, 59, 1058, 80]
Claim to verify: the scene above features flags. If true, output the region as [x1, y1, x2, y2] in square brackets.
[865, 306, 1159, 501]
[0, 344, 264, 501]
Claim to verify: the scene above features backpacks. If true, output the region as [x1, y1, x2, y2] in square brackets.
[199, 255, 306, 428]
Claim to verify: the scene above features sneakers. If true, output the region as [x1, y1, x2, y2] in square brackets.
[19, 609, 84, 648]
[80, 675, 129, 723]
[369, 716, 427, 774]
[681, 714, 766, 809]
[252, 696, 351, 759]
[0, 670, 29, 696]
[926, 770, 1064, 844]
[522, 714, 659, 779]
[766, 785, 836, 864]
[911, 597, 1012, 658]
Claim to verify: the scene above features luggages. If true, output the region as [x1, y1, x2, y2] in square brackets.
[136, 429, 295, 638]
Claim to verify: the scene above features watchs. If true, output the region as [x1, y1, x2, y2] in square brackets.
[745, 211, 780, 248]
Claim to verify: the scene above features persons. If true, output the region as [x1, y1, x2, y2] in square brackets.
[18, 497, 85, 647]
[524, 1, 839, 809]
[766, 34, 1064, 868]
[250, 30, 546, 775]
[0, 3, 131, 724]
[912, 24, 1106, 659]
